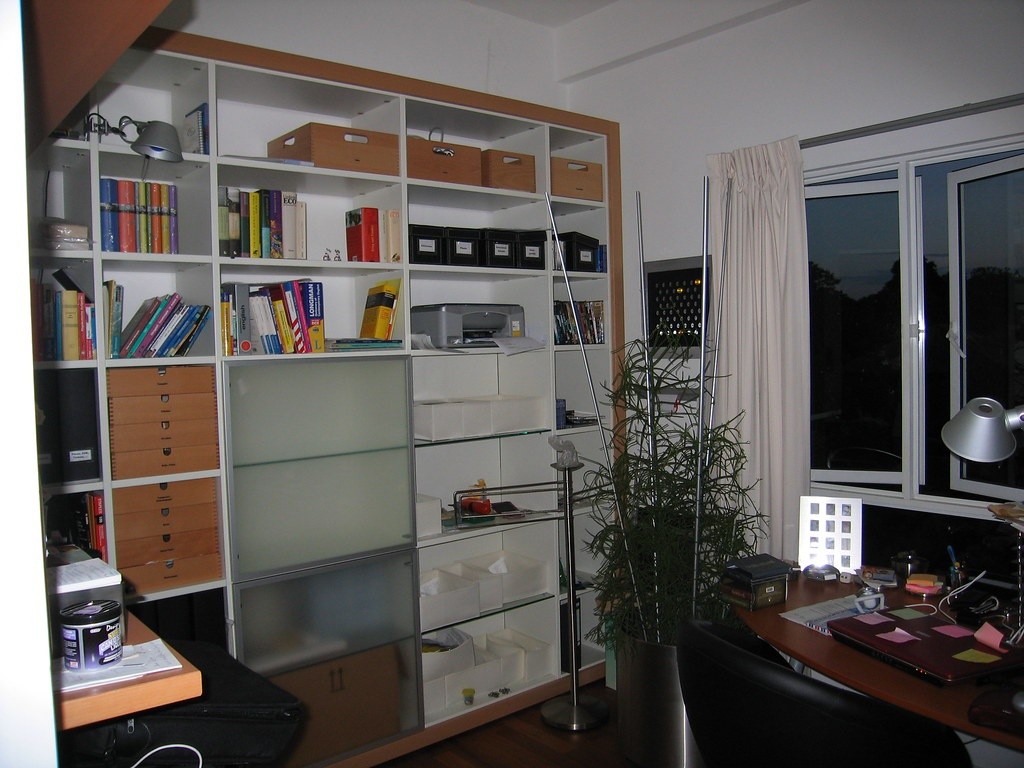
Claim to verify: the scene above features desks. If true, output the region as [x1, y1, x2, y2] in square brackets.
[46, 606, 203, 733]
[718, 570, 1024, 755]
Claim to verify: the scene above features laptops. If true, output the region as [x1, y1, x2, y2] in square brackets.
[827, 606, 1024, 688]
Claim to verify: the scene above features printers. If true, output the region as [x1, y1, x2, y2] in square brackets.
[410, 303, 526, 349]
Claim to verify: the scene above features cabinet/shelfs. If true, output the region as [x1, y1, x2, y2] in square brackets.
[24, 26, 633, 768]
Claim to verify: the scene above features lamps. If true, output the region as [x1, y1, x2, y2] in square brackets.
[67, 95, 186, 165]
[939, 395, 1024, 467]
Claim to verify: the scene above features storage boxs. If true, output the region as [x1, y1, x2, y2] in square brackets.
[267, 122, 605, 204]
[265, 642, 403, 768]
[106, 365, 227, 601]
[45, 542, 129, 661]
[409, 222, 600, 273]
[415, 492, 442, 542]
[413, 392, 552, 440]
[418, 548, 549, 725]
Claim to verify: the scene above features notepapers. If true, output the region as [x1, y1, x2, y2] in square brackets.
[906, 575, 939, 595]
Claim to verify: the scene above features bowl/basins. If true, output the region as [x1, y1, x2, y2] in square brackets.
[890, 556, 928, 578]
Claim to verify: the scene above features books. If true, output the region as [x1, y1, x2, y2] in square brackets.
[491, 502, 520, 514]
[565, 409, 605, 428]
[346, 208, 400, 263]
[30, 279, 96, 361]
[220, 279, 403, 356]
[100, 178, 179, 254]
[76, 494, 108, 563]
[102, 279, 212, 359]
[553, 300, 604, 344]
[217, 186, 308, 260]
[183, 103, 209, 154]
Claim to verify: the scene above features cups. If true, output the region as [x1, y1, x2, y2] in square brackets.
[555, 241, 566, 270]
[595, 245, 606, 272]
[556, 399, 566, 429]
[59, 600, 123, 671]
[944, 567, 968, 596]
[566, 410, 574, 428]
[463, 689, 475, 706]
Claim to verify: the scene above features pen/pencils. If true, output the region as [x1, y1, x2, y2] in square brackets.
[947, 545, 957, 566]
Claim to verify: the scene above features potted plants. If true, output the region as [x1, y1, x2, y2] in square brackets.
[589, 331, 767, 768]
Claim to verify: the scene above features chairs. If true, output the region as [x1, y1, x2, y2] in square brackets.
[676, 617, 975, 768]
